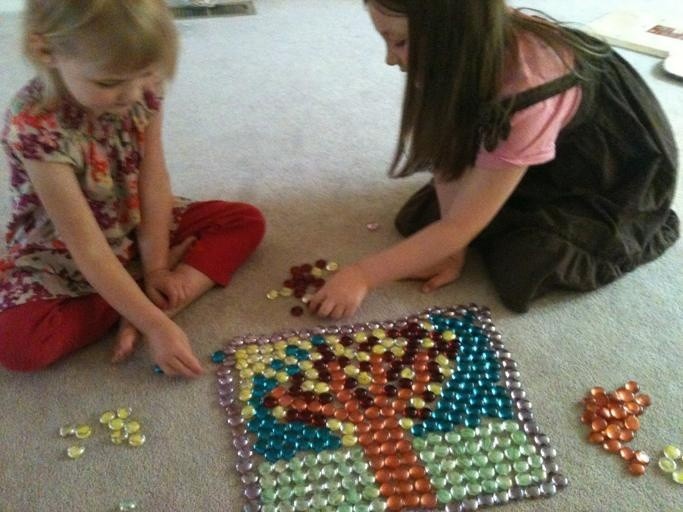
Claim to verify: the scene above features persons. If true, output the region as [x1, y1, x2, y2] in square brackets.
[0, 0, 265, 378]
[309, 0, 680, 319]
